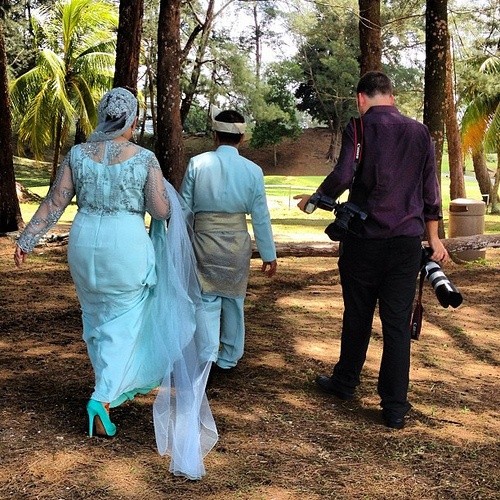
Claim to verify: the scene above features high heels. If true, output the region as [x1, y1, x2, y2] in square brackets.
[86, 398, 118, 438]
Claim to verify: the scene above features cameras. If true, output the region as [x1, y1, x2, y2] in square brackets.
[421, 247, 463, 308]
[303, 193, 368, 241]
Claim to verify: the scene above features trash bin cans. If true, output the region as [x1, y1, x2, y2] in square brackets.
[448, 198, 486, 264]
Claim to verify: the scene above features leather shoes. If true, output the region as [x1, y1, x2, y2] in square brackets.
[316, 375, 355, 399]
[382, 409, 405, 429]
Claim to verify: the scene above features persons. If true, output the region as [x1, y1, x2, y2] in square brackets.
[11, 86, 171, 437]
[293, 71, 447, 430]
[180, 110, 278, 377]
